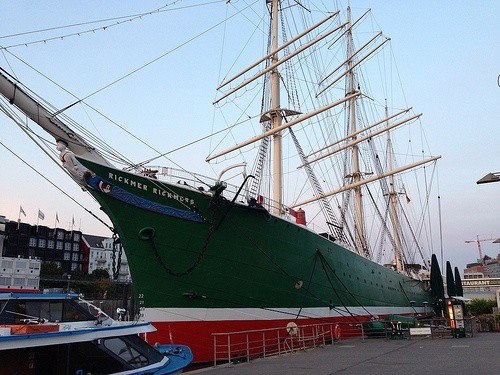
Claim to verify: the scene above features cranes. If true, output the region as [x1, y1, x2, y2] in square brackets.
[463, 234, 496, 260]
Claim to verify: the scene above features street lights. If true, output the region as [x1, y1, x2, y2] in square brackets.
[67, 275, 71, 292]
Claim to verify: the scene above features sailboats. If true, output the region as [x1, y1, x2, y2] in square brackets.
[0, 10, 443, 365]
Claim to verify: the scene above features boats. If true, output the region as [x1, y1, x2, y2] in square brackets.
[0, 278, 194, 375]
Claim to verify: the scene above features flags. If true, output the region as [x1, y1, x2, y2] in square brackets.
[20, 208, 26, 216]
[38, 211, 46, 220]
[56, 215, 59, 222]
